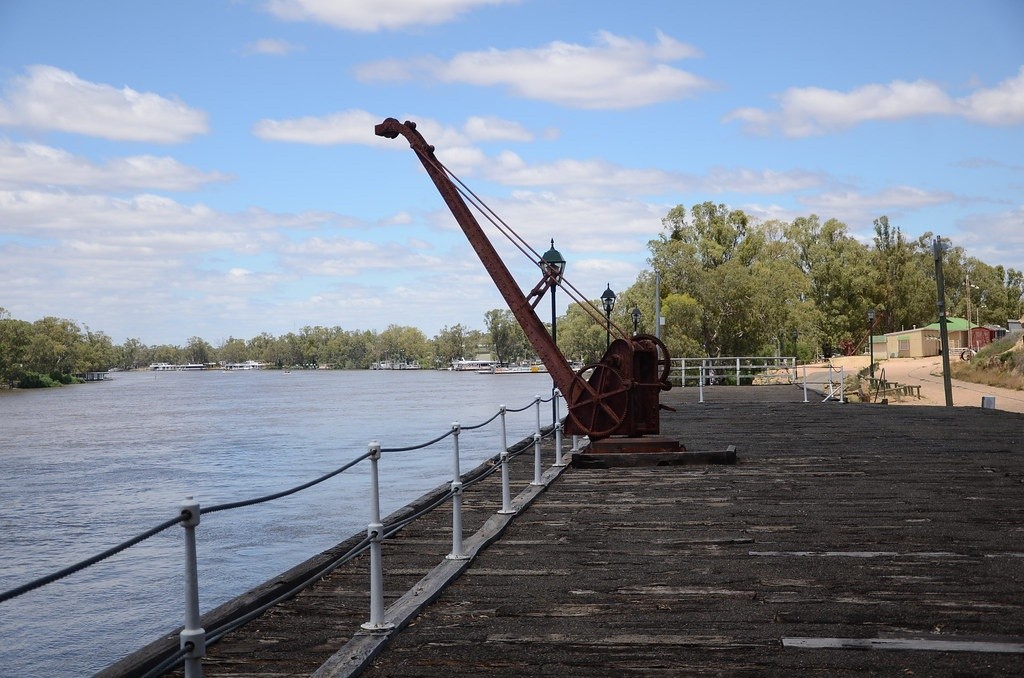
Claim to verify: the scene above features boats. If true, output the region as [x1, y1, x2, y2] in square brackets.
[450, 360, 548, 374]
[567, 360, 594, 372]
[179, 361, 263, 371]
[146, 362, 178, 371]
[370, 349, 421, 370]
[86, 372, 110, 381]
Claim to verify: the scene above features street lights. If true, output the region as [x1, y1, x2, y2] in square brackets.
[601, 283, 617, 350]
[631, 303, 642, 336]
[540, 238, 566, 438]
[867, 306, 876, 390]
